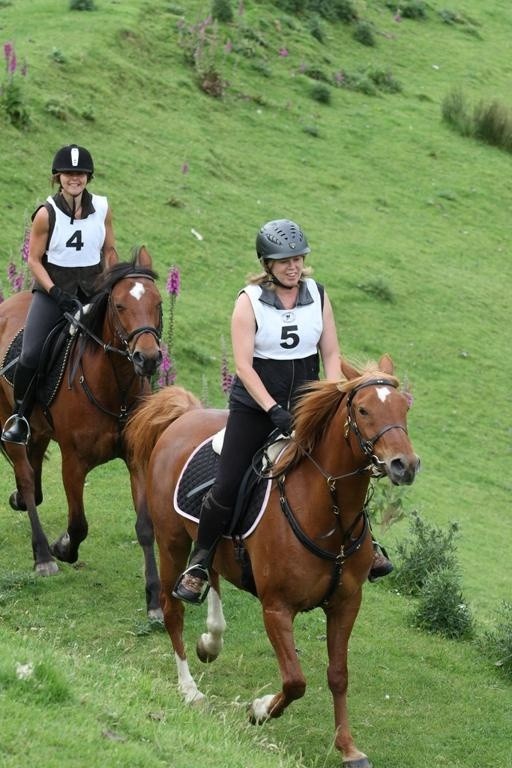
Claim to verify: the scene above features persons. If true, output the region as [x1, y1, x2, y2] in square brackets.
[4, 143, 119, 442]
[177, 219, 395, 603]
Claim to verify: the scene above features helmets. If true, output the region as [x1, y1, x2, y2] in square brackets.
[256, 219, 311, 260]
[52, 143, 93, 174]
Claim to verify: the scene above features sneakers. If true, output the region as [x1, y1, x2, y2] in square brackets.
[177, 568, 208, 602]
[370, 552, 394, 577]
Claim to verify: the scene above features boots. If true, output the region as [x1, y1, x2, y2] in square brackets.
[1, 361, 40, 444]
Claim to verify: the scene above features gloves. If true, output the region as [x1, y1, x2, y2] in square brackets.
[48, 285, 79, 313]
[266, 403, 292, 437]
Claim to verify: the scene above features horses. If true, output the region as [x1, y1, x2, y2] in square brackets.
[120, 355, 420, 768]
[0, 244, 167, 577]
